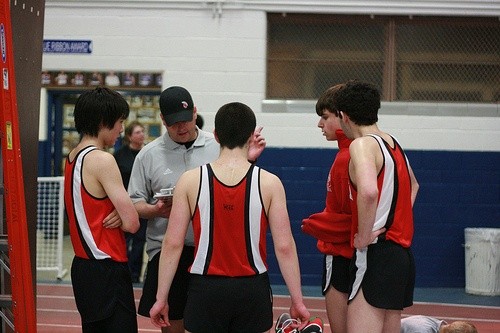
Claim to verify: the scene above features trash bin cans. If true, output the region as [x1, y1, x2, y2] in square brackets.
[463, 227, 499, 295]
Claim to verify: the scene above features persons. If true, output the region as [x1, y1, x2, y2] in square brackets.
[298, 84, 357, 333]
[400, 314, 478, 333]
[110, 121, 147, 284]
[336, 79, 420, 333]
[64, 86, 140, 333]
[127, 86, 266, 333]
[149, 102, 311, 333]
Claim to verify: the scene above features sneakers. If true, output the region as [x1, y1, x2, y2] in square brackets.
[301, 316, 323, 333]
[276, 311, 298, 333]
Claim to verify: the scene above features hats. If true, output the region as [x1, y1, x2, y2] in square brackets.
[159, 86, 194, 126]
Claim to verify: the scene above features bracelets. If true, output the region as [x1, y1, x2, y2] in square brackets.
[246, 157, 259, 165]
